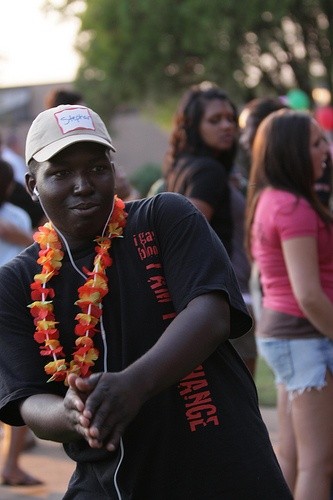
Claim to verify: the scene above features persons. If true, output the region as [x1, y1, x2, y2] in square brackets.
[0, 82, 333, 500]
[0, 104, 294, 500]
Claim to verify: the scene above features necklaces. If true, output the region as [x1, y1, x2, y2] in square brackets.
[28, 195, 128, 388]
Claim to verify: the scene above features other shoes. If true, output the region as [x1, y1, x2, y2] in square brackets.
[0, 471, 46, 497]
[22, 436, 38, 451]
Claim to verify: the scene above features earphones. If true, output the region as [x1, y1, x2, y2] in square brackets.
[34, 187, 40, 196]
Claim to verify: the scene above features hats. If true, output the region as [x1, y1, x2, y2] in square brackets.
[23, 104, 117, 167]
